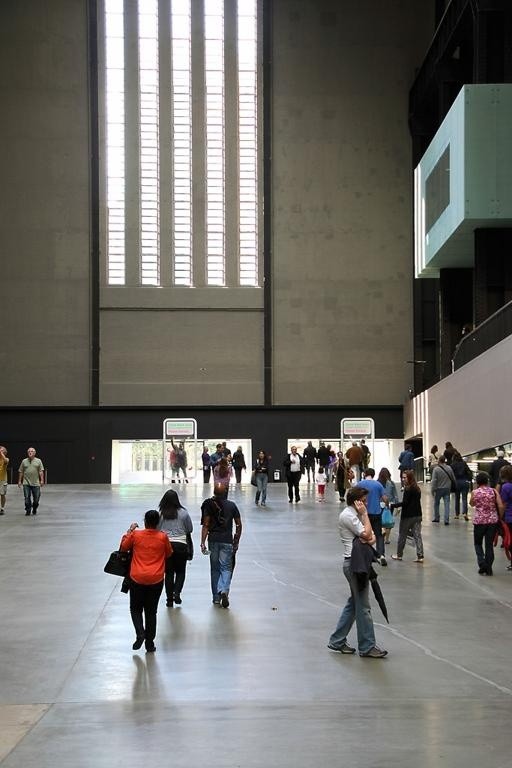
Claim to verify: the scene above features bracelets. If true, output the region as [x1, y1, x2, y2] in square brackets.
[128, 527, 133, 531]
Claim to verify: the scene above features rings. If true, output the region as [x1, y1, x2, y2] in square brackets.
[359, 503, 361, 505]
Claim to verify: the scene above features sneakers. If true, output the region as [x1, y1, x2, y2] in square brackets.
[359, 647, 388, 657]
[392, 554, 402, 560]
[133, 633, 143, 650]
[432, 520, 439, 522]
[167, 593, 183, 607]
[328, 642, 355, 654]
[213, 592, 228, 608]
[454, 516, 460, 519]
[464, 515, 468, 521]
[413, 560, 424, 563]
[378, 555, 387, 566]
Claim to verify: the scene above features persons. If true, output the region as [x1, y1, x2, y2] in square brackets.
[200, 480, 243, 608]
[399, 444, 415, 490]
[469, 451, 512, 575]
[355, 467, 424, 566]
[170, 439, 188, 484]
[231, 446, 246, 483]
[18, 447, 47, 515]
[149, 489, 194, 608]
[201, 442, 233, 500]
[303, 439, 370, 503]
[120, 510, 174, 652]
[0, 445, 11, 514]
[428, 441, 473, 526]
[283, 446, 307, 503]
[253, 450, 269, 506]
[328, 487, 392, 658]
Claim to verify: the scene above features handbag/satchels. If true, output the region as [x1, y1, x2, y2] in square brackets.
[450, 481, 455, 494]
[251, 471, 256, 486]
[104, 552, 130, 578]
[348, 470, 354, 479]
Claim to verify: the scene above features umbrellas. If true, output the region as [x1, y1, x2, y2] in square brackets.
[366, 564, 390, 623]
